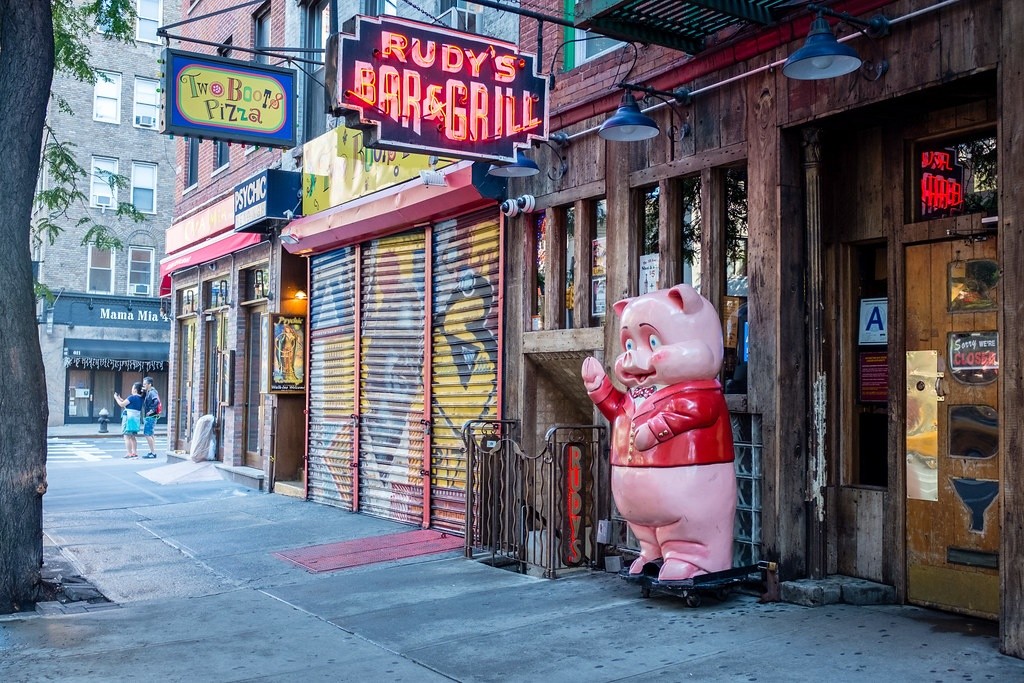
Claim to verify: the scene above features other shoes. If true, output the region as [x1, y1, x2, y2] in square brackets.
[121, 453, 138, 460]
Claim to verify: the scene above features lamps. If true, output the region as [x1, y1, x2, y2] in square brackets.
[782, 4, 890, 79]
[254, 270, 273, 300]
[420, 156, 457, 187]
[186, 290, 200, 316]
[279, 210, 302, 246]
[160, 297, 174, 322]
[87, 298, 93, 310]
[488, 132, 568, 181]
[125, 299, 133, 311]
[219, 280, 234, 309]
[597, 83, 690, 142]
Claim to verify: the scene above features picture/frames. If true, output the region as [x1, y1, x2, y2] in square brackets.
[267, 312, 306, 394]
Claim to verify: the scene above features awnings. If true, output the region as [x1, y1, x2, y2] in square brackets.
[63, 337, 170, 372]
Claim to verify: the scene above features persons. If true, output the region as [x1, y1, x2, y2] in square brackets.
[114, 382, 146, 458]
[143, 377, 162, 458]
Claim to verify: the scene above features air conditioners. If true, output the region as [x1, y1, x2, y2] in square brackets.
[96, 194, 112, 208]
[134, 284, 150, 295]
[138, 115, 153, 126]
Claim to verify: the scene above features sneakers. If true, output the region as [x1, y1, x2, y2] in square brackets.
[142, 452, 156, 459]
